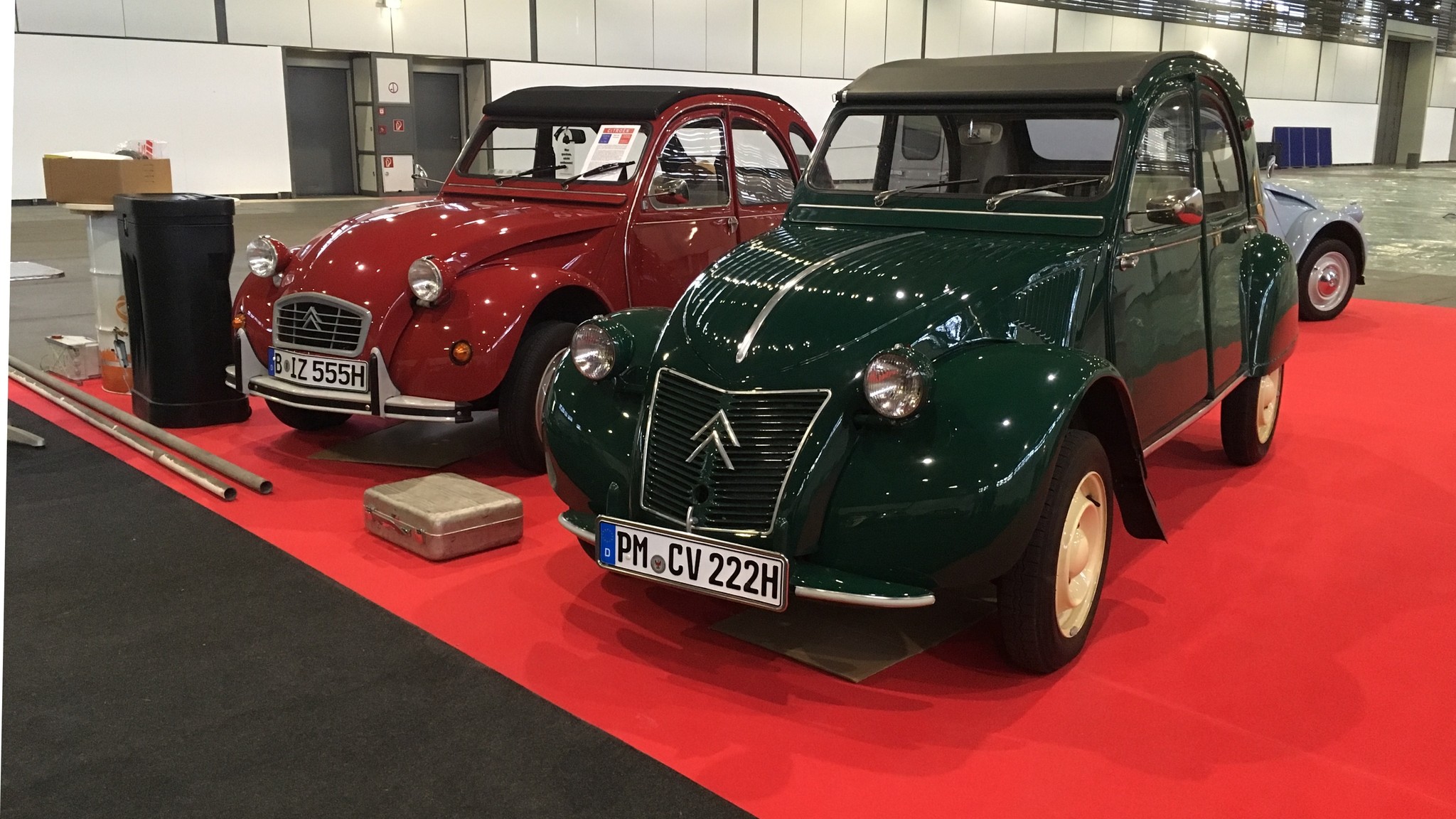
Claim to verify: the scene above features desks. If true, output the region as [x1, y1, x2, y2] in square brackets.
[8, 261, 65, 447]
[65, 203, 131, 359]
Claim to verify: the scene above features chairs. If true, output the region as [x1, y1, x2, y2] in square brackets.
[652, 168, 796, 207]
[983, 174, 1192, 229]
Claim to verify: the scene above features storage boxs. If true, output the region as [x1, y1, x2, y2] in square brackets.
[45, 334, 101, 380]
[43, 157, 172, 205]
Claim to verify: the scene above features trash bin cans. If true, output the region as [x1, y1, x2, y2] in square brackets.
[112, 192, 252, 427]
[1256, 142, 1282, 169]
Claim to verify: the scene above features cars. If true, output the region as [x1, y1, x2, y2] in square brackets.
[535, 48, 1297, 677]
[225, 84, 836, 475]
[1261, 153, 1367, 320]
